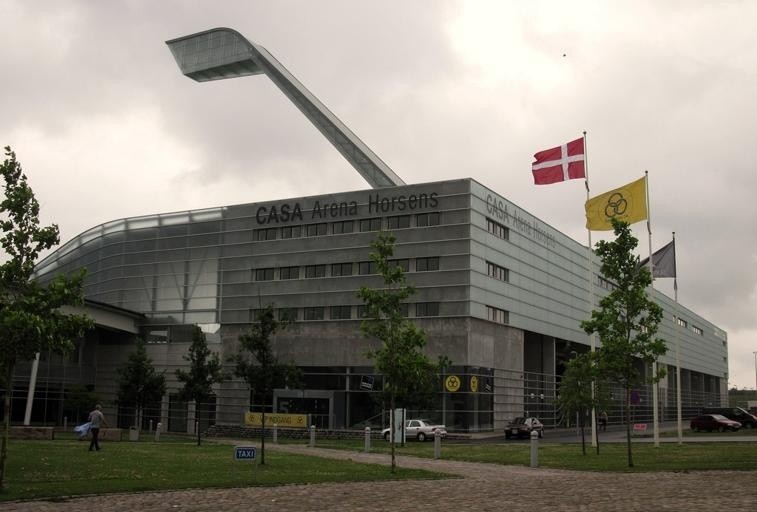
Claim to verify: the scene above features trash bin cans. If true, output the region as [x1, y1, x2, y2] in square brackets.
[128, 425, 139, 441]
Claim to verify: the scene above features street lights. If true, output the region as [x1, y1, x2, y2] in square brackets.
[751, 350, 756, 390]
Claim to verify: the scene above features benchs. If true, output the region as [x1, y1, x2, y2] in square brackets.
[10, 426, 123, 442]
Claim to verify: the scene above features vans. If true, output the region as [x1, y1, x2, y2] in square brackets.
[702, 406, 756, 428]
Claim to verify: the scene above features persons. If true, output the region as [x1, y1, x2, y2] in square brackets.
[598, 410, 608, 432]
[86, 403, 110, 452]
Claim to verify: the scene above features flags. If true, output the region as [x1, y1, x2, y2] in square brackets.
[583, 175, 647, 232]
[530, 136, 584, 186]
[637, 238, 674, 278]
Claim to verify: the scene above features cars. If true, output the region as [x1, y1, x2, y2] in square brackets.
[503, 416, 544, 439]
[689, 412, 742, 433]
[380, 418, 447, 442]
